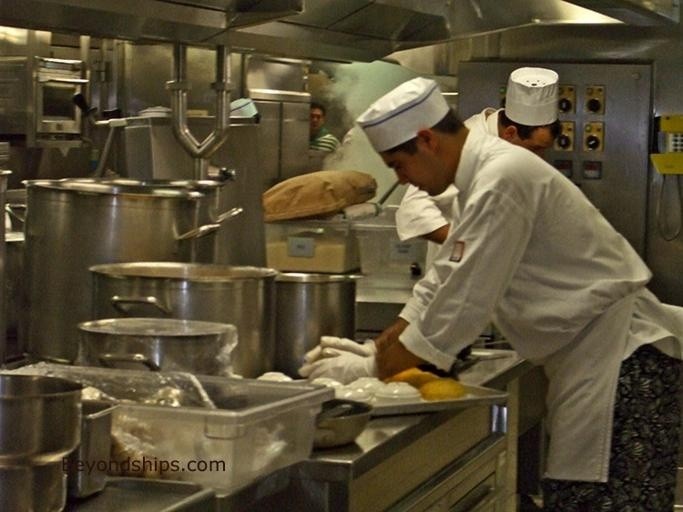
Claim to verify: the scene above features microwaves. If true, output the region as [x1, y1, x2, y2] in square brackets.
[34, 66, 82, 135]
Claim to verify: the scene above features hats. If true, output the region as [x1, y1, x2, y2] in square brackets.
[355, 76, 452, 153]
[505, 66, 559, 127]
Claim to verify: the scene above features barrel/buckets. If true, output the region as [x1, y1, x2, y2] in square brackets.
[21, 174, 364, 382]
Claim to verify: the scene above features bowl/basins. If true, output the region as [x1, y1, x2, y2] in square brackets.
[0, 370, 90, 512]
[309, 397, 374, 451]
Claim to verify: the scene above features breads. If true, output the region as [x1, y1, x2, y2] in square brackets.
[390, 367, 468, 403]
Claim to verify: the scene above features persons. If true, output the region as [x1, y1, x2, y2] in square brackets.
[310, 102, 341, 153]
[394, 60, 563, 274]
[298, 75, 683, 511]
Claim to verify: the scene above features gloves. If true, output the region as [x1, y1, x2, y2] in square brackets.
[303, 334, 377, 365]
[297, 346, 378, 385]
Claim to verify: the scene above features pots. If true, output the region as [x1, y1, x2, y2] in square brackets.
[274, 270, 365, 377]
[2, 175, 242, 368]
[74, 259, 278, 377]
